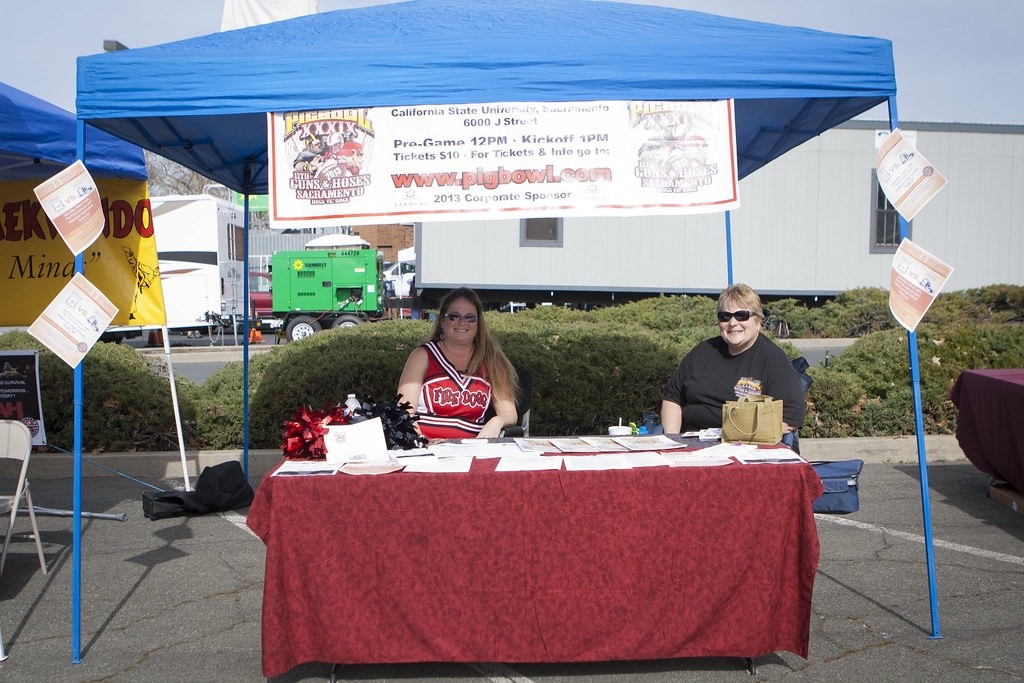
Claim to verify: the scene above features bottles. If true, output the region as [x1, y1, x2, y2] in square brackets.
[344, 393, 362, 417]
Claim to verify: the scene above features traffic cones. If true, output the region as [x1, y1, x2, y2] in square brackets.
[247, 327, 266, 344]
[144, 329, 163, 347]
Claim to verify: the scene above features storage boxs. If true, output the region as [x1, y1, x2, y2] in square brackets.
[989, 483, 1024, 516]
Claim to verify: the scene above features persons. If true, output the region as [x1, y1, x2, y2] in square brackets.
[396, 287, 518, 438]
[660, 284, 805, 434]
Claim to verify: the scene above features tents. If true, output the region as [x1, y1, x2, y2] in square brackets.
[0, 81, 191, 491]
[72, 0, 942, 664]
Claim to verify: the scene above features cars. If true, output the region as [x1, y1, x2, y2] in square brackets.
[249, 272, 273, 319]
[383, 260, 416, 298]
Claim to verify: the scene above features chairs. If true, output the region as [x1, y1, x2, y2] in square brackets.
[1, 418, 48, 588]
[482, 367, 535, 438]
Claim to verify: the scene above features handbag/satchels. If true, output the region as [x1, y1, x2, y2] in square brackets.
[804, 457, 863, 513]
[722, 395, 783, 446]
[196, 460, 255, 512]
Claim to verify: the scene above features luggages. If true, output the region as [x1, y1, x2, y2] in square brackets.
[141, 489, 207, 521]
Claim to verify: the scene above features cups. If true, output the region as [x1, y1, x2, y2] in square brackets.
[608, 426, 632, 435]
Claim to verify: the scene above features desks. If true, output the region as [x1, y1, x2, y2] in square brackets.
[248, 431, 824, 677]
[951, 369, 1024, 494]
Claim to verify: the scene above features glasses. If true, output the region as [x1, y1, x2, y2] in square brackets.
[444, 312, 478, 322]
[717, 310, 760, 322]
